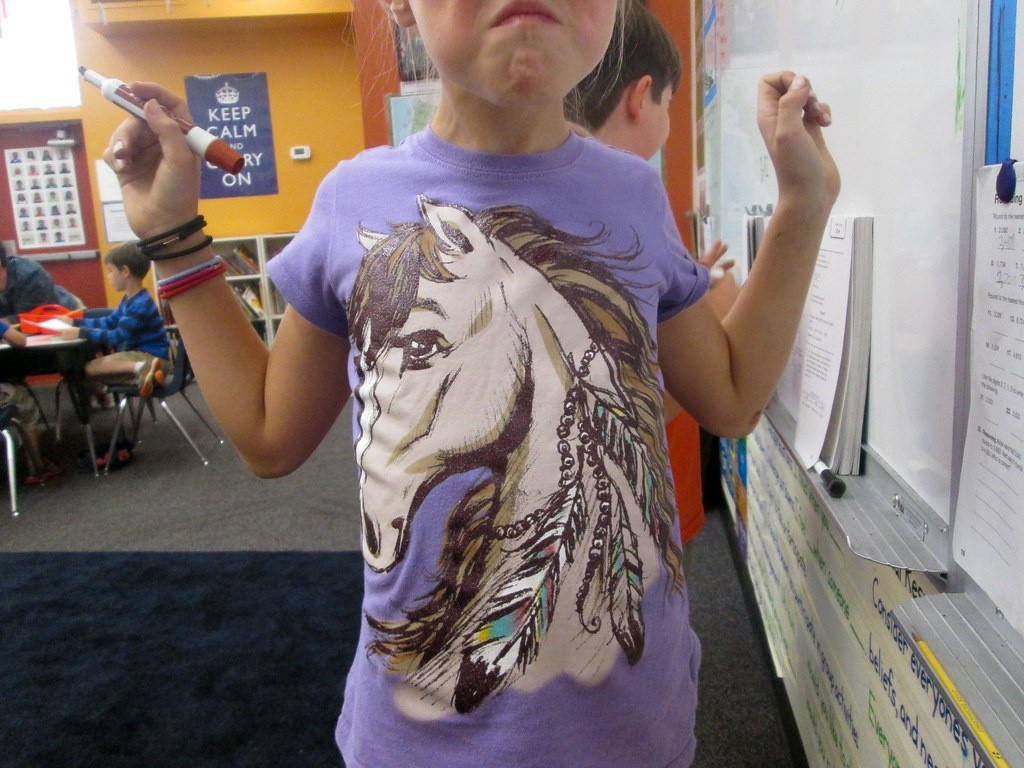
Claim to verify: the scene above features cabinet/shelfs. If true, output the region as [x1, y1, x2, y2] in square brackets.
[152, 235, 272, 343]
[259, 232, 298, 345]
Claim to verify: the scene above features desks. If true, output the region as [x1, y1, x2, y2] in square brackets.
[0, 335, 136, 477]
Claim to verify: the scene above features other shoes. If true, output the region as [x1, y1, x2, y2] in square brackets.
[136, 357, 162, 397]
[20, 463, 64, 485]
[77, 439, 130, 475]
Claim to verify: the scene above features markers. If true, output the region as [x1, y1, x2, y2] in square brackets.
[78, 66, 245, 175]
[814, 459, 847, 498]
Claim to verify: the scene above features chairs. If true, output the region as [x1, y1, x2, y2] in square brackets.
[17, 304, 85, 441]
[105, 335, 223, 473]
[0, 417, 46, 518]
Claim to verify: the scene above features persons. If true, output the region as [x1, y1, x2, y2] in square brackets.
[62, 240, 174, 467]
[564, 0, 736, 579]
[11, 149, 79, 244]
[0, 240, 115, 408]
[0, 320, 62, 485]
[101, 0, 838, 767]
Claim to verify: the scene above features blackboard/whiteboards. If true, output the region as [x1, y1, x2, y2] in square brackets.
[712, 0, 990, 574]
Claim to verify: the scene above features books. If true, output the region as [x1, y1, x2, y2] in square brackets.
[220, 243, 260, 276]
[743, 215, 874, 476]
[229, 282, 265, 319]
[270, 282, 288, 315]
[160, 299, 179, 367]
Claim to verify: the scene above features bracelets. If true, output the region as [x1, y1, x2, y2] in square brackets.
[137, 215, 225, 298]
